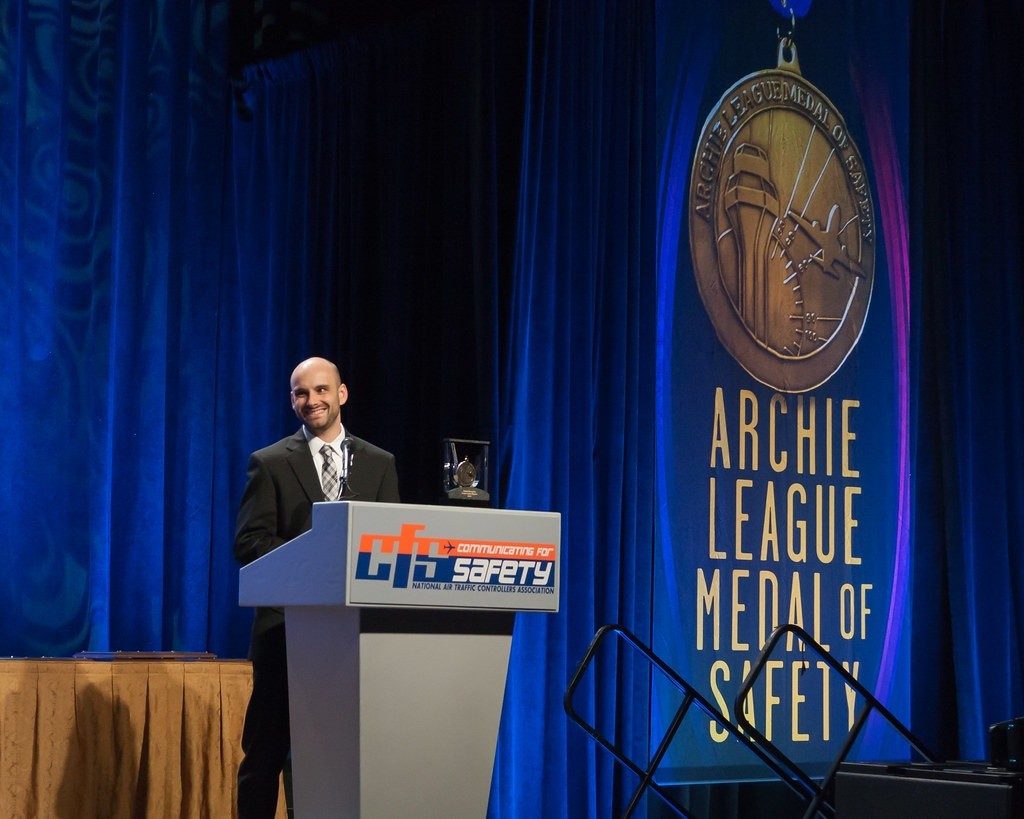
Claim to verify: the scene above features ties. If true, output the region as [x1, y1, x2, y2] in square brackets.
[319, 445, 340, 502]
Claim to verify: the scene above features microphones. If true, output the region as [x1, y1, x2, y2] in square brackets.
[340, 437, 356, 487]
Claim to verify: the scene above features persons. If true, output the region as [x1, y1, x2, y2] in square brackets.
[231, 357, 402, 819]
